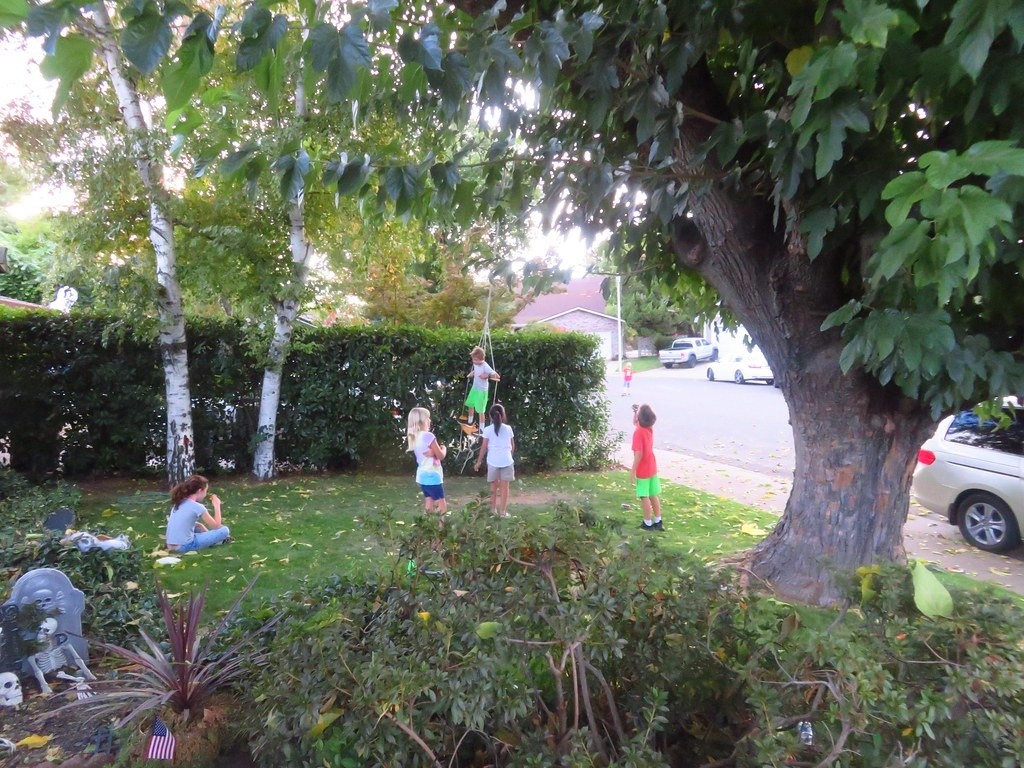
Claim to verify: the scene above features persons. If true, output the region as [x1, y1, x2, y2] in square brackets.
[405, 407, 447, 529]
[474, 404, 516, 519]
[632, 404, 664, 532]
[457, 346, 500, 437]
[166, 477, 230, 553]
[621, 362, 633, 397]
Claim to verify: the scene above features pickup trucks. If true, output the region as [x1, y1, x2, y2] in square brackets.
[659, 337, 719, 368]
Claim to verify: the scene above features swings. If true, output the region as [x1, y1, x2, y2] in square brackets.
[456, 284, 500, 438]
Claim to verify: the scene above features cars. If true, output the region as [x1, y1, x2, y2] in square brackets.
[912, 403, 1024, 552]
[706, 356, 774, 385]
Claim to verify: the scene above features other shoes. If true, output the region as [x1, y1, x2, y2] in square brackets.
[489, 512, 499, 519]
[441, 511, 453, 516]
[210, 536, 234, 547]
[653, 520, 665, 530]
[500, 513, 511, 519]
[634, 521, 655, 530]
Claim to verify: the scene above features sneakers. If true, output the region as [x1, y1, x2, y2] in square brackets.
[456, 418, 475, 427]
[472, 428, 485, 436]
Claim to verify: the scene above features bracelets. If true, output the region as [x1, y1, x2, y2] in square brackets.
[488, 373, 490, 378]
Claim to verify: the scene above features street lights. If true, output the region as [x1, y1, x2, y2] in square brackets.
[615, 272, 622, 376]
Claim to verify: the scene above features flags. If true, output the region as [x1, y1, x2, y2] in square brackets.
[146, 716, 176, 760]
